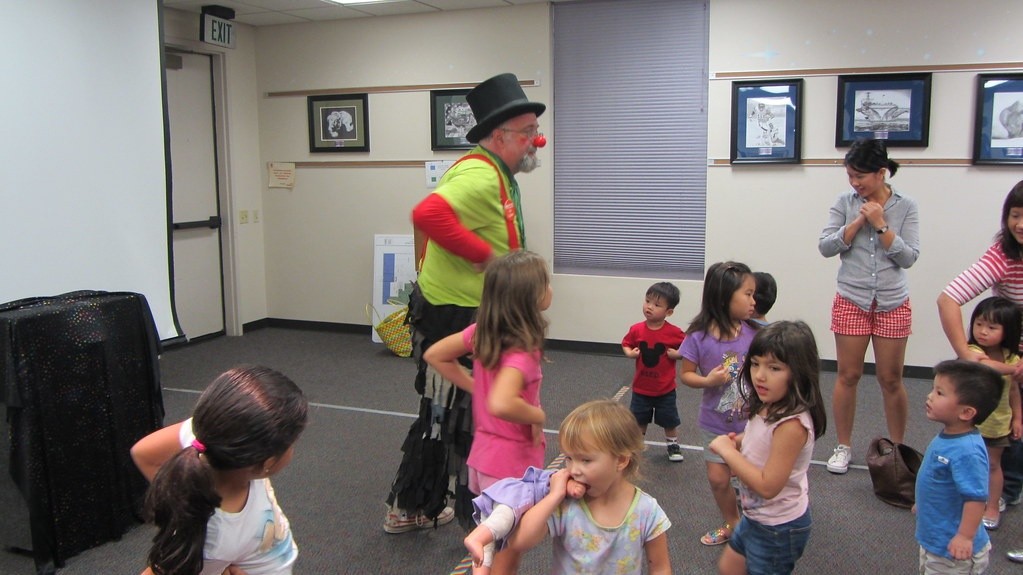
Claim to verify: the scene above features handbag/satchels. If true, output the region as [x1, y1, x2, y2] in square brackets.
[866, 436, 924, 508]
[365, 300, 414, 357]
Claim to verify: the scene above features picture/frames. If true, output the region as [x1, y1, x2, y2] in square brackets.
[730, 78, 803, 164]
[430, 89, 477, 151]
[307, 93, 370, 153]
[973, 73, 1023, 167]
[835, 72, 932, 147]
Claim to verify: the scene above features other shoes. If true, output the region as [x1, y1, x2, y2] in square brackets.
[982, 513, 1000, 530]
[1006, 549, 1023, 563]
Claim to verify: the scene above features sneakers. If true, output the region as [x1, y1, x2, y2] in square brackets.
[827, 444, 851, 473]
[383, 506, 454, 534]
[986, 494, 1021, 512]
[667, 444, 683, 460]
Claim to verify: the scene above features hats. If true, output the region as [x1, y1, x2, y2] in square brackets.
[466, 73, 546, 143]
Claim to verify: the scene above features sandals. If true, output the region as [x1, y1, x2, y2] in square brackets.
[701, 520, 734, 545]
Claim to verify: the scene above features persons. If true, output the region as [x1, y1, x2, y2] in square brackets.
[957, 296, 1022, 530]
[911, 360, 1006, 575]
[707, 320, 827, 575]
[129, 363, 308, 575]
[383, 73, 547, 533]
[463, 465, 587, 575]
[935, 180, 1023, 505]
[621, 282, 686, 460]
[422, 247, 553, 575]
[677, 261, 766, 546]
[748, 272, 777, 328]
[818, 136, 920, 475]
[507, 397, 673, 575]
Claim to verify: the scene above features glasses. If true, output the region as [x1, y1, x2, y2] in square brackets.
[500, 128, 538, 139]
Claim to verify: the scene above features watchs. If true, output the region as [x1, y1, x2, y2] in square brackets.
[876, 225, 889, 234]
